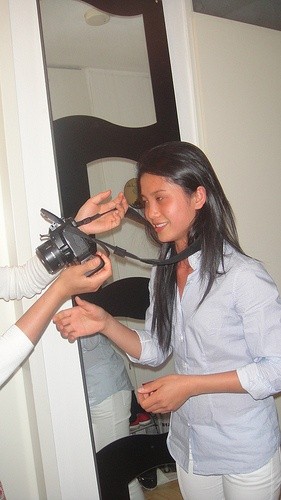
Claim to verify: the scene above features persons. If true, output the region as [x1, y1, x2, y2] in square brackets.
[51, 141, 281, 500]
[0, 189, 128, 387]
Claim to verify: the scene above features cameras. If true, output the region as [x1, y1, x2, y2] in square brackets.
[35, 216, 105, 275]
